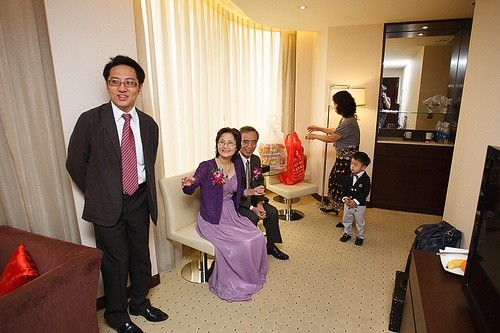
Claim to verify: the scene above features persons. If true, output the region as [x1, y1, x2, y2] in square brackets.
[182, 126, 290, 302]
[305, 91, 361, 227]
[340, 152, 371, 245]
[65, 55, 169, 333]
[378, 85, 391, 136]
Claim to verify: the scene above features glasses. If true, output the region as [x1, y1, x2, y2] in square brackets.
[106, 80, 140, 87]
[216, 140, 237, 147]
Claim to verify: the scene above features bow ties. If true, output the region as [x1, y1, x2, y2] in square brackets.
[352, 174, 358, 179]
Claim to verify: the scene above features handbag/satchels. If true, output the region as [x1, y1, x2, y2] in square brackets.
[414, 220, 462, 253]
[280, 132, 306, 185]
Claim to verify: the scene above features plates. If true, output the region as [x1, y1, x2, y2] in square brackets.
[439, 247, 469, 276]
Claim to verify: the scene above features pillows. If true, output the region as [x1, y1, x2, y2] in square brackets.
[0, 243, 39, 298]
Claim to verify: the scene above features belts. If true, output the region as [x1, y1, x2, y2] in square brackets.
[121, 182, 150, 195]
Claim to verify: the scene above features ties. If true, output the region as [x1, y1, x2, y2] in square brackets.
[121, 113, 139, 196]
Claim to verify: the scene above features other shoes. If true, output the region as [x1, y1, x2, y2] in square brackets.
[355, 236, 364, 246]
[339, 233, 352, 242]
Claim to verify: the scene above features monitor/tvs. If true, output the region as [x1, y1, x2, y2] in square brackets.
[461, 144, 500, 333]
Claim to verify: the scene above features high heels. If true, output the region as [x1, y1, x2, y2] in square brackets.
[320, 207, 339, 215]
[336, 222, 345, 227]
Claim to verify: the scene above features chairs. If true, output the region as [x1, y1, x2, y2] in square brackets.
[266, 175, 318, 221]
[158, 170, 215, 284]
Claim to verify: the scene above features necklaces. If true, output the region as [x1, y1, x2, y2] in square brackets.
[218, 159, 233, 180]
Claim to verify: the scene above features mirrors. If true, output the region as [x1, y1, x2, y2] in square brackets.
[377, 28, 461, 137]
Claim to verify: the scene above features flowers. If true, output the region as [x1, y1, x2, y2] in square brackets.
[211, 168, 226, 186]
[252, 167, 262, 179]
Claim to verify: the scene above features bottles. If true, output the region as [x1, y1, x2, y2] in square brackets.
[435, 123, 450, 143]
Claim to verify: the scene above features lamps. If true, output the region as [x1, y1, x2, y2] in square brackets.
[320, 85, 366, 207]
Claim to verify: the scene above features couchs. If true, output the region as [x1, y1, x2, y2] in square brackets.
[0, 225, 104, 333]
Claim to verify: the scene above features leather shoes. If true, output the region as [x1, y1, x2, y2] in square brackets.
[267, 246, 289, 260]
[116, 320, 144, 333]
[130, 305, 169, 322]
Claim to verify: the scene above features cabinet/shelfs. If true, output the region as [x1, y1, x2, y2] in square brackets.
[370, 143, 454, 215]
[400, 249, 478, 333]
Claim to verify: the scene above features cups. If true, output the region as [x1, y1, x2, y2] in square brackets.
[426, 133, 434, 139]
[404, 132, 412, 138]
